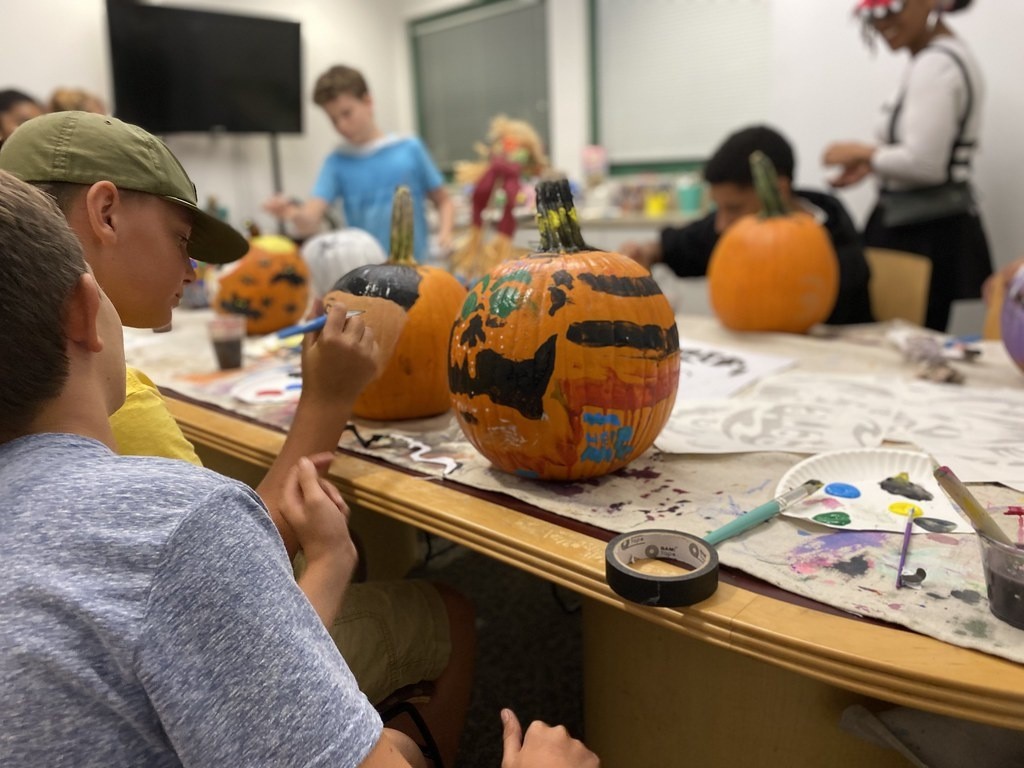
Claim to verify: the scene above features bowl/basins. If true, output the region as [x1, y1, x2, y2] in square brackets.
[970, 505, 1024, 632]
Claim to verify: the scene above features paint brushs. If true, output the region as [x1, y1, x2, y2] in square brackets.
[701, 479, 826, 542]
[896, 506, 915, 589]
[276, 310, 368, 339]
[933, 464, 1016, 549]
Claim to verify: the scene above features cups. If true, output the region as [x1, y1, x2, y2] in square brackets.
[206, 314, 243, 368]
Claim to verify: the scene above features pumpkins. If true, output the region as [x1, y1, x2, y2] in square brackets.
[211, 147, 1024, 477]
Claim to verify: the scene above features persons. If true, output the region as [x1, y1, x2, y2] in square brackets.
[612, 124, 882, 329]
[0, 88, 45, 148]
[820, 0, 996, 332]
[0, 106, 388, 564]
[1, 168, 600, 768]
[265, 65, 459, 269]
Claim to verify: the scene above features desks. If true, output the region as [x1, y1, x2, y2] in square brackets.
[106, 296, 1023, 768]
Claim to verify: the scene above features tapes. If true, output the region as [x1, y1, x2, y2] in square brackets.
[604, 529, 719, 610]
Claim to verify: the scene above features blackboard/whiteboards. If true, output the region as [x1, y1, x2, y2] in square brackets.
[585, 0, 773, 178]
[103, 0, 302, 139]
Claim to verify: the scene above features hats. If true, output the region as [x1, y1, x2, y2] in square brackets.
[0, 111, 251, 264]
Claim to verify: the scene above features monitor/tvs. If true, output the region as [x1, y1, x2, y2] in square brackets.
[105, 0, 305, 139]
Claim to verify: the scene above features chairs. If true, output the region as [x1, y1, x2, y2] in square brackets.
[861, 244, 934, 327]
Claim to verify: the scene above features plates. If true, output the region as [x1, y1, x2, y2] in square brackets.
[774, 448, 978, 534]
[230, 370, 303, 404]
